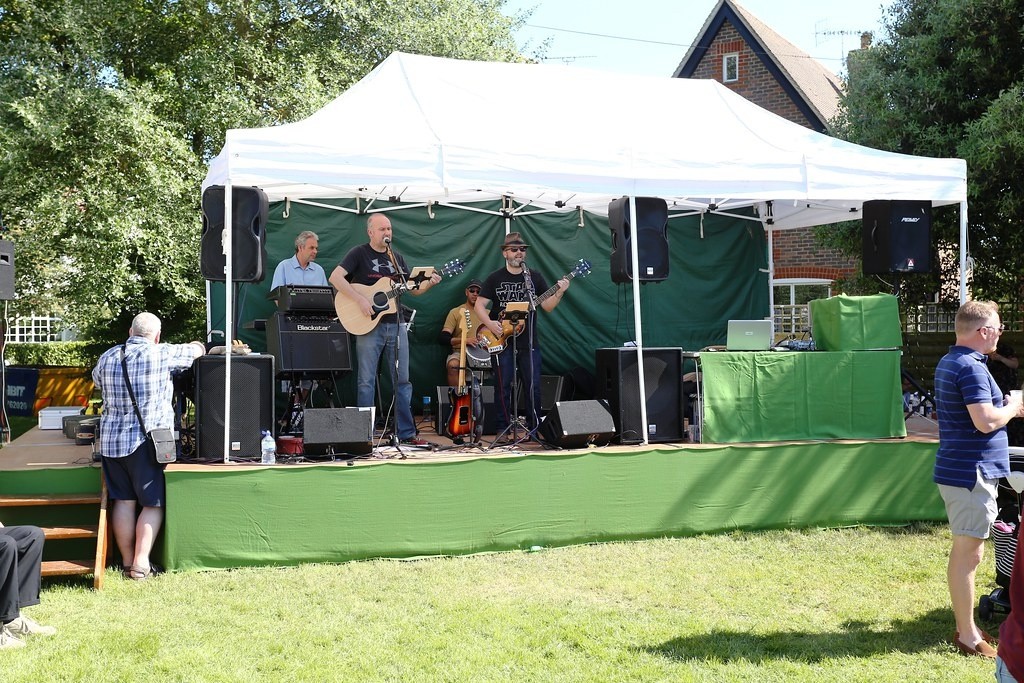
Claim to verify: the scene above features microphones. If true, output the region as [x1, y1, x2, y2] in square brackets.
[384, 237, 392, 244]
[519, 261, 528, 273]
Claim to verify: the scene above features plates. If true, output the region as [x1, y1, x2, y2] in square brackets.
[279, 436, 295, 438]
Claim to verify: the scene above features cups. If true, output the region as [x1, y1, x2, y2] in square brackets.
[1009, 390, 1023, 405]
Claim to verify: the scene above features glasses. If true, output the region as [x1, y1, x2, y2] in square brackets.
[505, 246, 526, 252]
[977, 326, 1002, 333]
[468, 288, 479, 294]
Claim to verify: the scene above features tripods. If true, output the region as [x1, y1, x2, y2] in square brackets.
[373, 245, 434, 458]
[489, 324, 547, 448]
[428, 365, 488, 454]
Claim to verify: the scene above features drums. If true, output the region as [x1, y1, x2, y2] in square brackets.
[465, 345, 492, 367]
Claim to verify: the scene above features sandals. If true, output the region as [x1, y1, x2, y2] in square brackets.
[124, 570, 131, 576]
[130, 561, 165, 580]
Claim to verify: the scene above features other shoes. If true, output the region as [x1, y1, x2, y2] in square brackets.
[530, 432, 539, 442]
[956, 639, 997, 658]
[403, 436, 428, 445]
[955, 631, 998, 643]
[496, 432, 508, 443]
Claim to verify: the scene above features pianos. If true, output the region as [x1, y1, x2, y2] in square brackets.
[241, 317, 367, 438]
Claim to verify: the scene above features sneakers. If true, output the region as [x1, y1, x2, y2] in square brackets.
[0, 626, 26, 649]
[4, 616, 57, 636]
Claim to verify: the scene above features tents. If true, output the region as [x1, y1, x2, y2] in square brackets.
[196, 50, 969, 453]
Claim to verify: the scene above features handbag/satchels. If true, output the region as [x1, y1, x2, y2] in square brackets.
[148, 429, 178, 467]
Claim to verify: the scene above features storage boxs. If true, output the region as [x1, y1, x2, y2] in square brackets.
[809, 291, 902, 352]
[37, 406, 86, 429]
[62, 415, 101, 438]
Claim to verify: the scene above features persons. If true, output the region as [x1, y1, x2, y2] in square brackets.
[86, 313, 207, 579]
[442, 276, 497, 421]
[0, 520, 59, 649]
[329, 213, 442, 448]
[269, 231, 330, 309]
[475, 231, 572, 443]
[933, 302, 1024, 683]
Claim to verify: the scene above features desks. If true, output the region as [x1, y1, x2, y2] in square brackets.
[681, 349, 907, 445]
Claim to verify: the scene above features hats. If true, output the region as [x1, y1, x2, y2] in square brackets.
[467, 279, 482, 289]
[501, 232, 530, 246]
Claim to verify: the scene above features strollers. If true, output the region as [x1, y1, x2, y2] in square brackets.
[978, 446, 1024, 622]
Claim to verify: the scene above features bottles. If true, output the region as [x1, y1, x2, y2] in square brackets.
[261, 430, 276, 464]
[423, 396, 431, 422]
[903, 391, 933, 420]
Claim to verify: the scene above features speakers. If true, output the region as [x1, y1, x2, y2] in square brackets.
[609, 197, 669, 282]
[544, 399, 617, 449]
[303, 407, 374, 458]
[594, 348, 685, 444]
[435, 385, 473, 436]
[200, 184, 269, 281]
[516, 374, 571, 416]
[264, 312, 353, 371]
[863, 199, 932, 273]
[194, 355, 276, 461]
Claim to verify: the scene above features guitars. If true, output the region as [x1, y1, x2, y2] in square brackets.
[334, 257, 467, 336]
[476, 258, 592, 355]
[445, 307, 477, 439]
